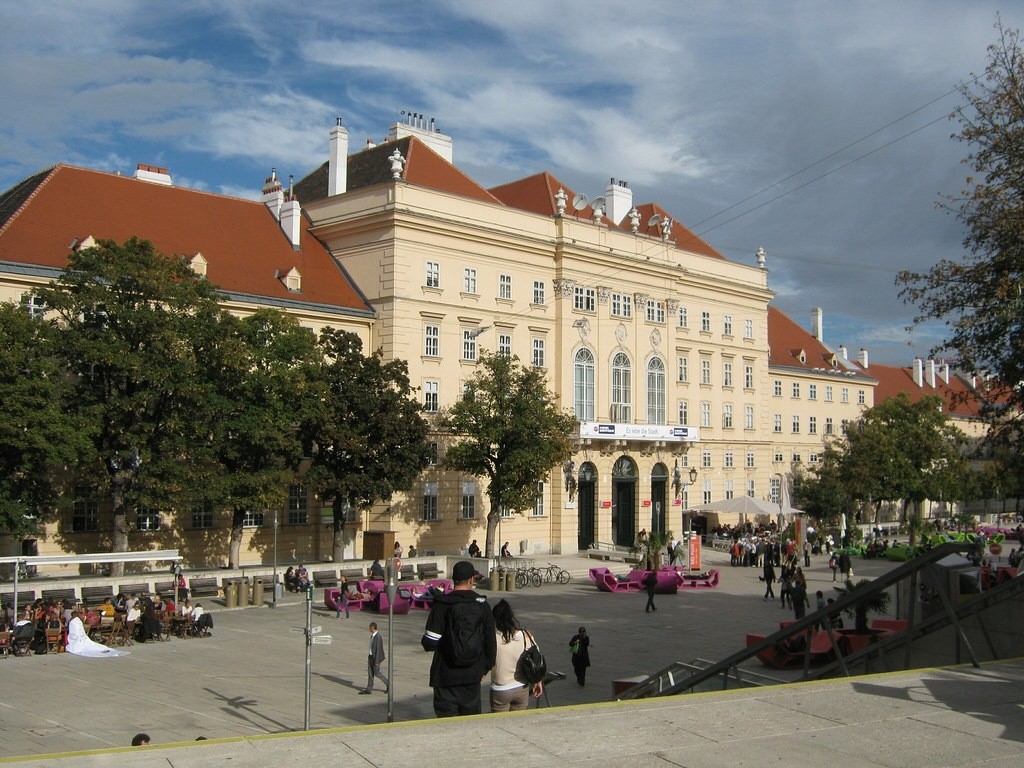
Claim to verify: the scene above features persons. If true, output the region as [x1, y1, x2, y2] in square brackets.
[469, 539, 479, 557]
[758, 550, 850, 632]
[490, 599, 543, 713]
[638, 528, 647, 544]
[421, 561, 496, 718]
[358, 622, 389, 694]
[667, 541, 686, 568]
[568, 627, 591, 689]
[863, 537, 897, 557]
[1009, 546, 1024, 569]
[98, 591, 174, 643]
[181, 601, 193, 616]
[1016, 524, 1022, 537]
[881, 526, 889, 537]
[1, 597, 99, 657]
[172, 574, 187, 601]
[284, 541, 445, 619]
[919, 534, 928, 544]
[191, 603, 204, 635]
[713, 519, 834, 567]
[642, 572, 658, 612]
[501, 542, 511, 557]
[856, 511, 861, 524]
[132, 732, 207, 746]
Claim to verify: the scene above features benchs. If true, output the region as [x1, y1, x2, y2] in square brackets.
[587, 549, 643, 563]
[188, 571, 309, 595]
[313, 562, 446, 586]
[0, 581, 190, 608]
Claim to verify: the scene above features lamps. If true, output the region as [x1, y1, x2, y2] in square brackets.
[569, 467, 593, 501]
[676, 467, 697, 496]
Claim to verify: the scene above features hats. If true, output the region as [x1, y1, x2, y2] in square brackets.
[453, 561, 479, 580]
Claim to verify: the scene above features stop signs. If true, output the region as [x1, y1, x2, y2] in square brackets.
[990, 543, 1002, 553]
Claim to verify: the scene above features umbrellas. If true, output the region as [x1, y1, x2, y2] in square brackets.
[680, 494, 806, 523]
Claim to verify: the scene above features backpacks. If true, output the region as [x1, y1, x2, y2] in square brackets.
[439, 598, 484, 668]
[829, 558, 836, 567]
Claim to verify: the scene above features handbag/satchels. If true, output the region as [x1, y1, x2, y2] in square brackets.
[522, 629, 546, 683]
[848, 568, 854, 577]
[569, 642, 579, 655]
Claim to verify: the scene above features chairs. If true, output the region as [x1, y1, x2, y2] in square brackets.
[746, 619, 907, 669]
[324, 580, 454, 615]
[0, 611, 211, 658]
[589, 566, 721, 592]
[833, 526, 1024, 588]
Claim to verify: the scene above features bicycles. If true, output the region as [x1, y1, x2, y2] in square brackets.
[489, 562, 570, 587]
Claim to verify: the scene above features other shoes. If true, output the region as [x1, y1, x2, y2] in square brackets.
[359, 691, 371, 694]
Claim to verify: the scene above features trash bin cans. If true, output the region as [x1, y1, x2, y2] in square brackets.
[499, 567, 507, 591]
[506, 568, 516, 591]
[253, 577, 264, 606]
[489, 567, 499, 592]
[237, 578, 249, 606]
[224, 580, 237, 607]
[500, 566, 506, 591]
